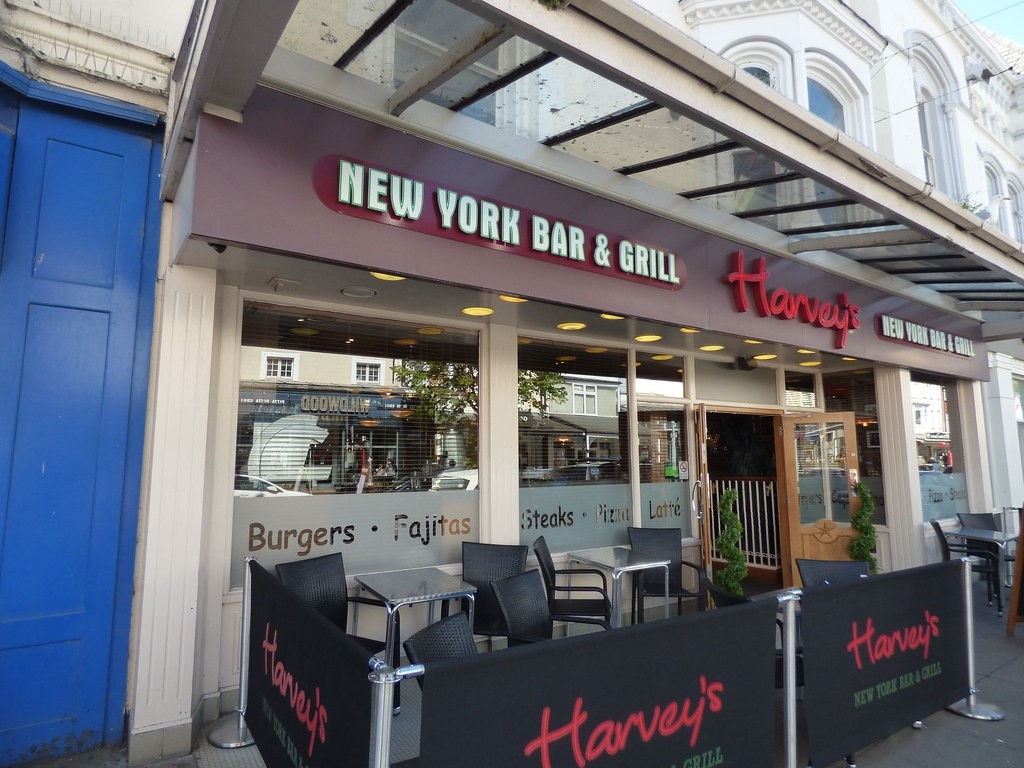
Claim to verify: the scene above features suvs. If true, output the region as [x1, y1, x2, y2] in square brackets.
[554, 457, 629, 483]
[426, 468, 481, 495]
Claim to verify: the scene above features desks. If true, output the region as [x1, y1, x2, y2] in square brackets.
[352, 567, 477, 667]
[944, 526, 1017, 607]
[565, 547, 671, 637]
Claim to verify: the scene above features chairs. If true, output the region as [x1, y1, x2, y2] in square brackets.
[929, 512, 1014, 615]
[275, 526, 869, 715]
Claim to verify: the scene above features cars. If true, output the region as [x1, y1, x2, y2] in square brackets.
[519, 467, 553, 487]
[235, 473, 313, 498]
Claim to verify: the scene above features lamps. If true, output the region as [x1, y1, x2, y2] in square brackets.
[737, 355, 758, 372]
